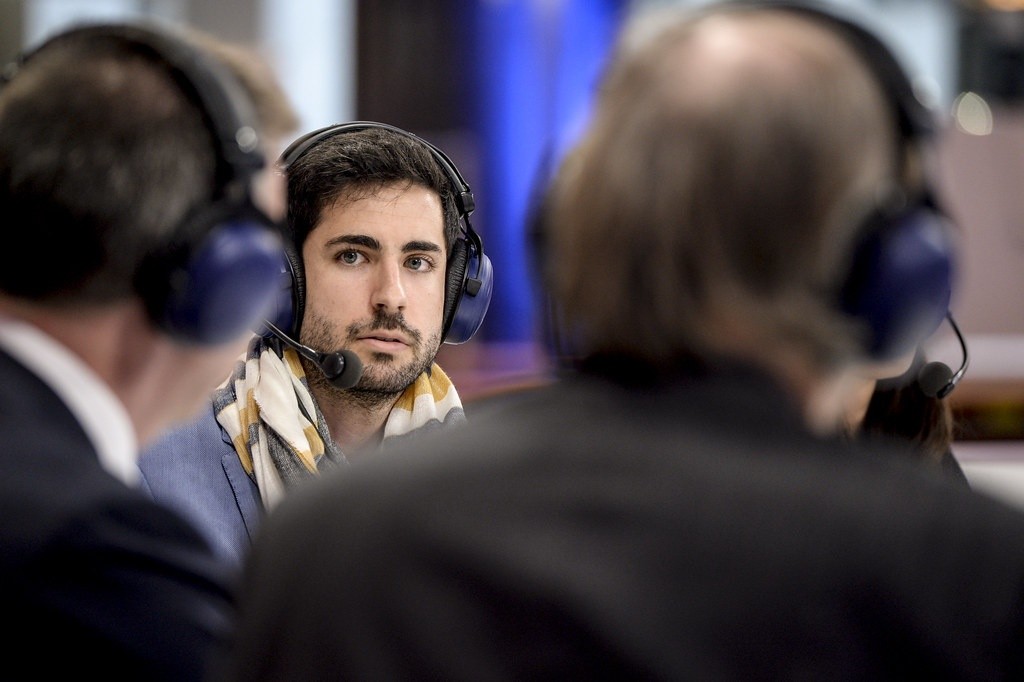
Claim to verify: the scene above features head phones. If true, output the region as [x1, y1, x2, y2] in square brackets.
[0, 19, 287, 347]
[250, 121, 494, 390]
[523, 0, 972, 400]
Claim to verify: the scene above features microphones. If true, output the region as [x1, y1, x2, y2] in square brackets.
[263, 319, 364, 390]
[915, 311, 973, 399]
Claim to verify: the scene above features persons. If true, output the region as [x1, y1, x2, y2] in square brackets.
[137, 118, 492, 551]
[0, 21, 297, 682]
[235, 0, 1024, 682]
